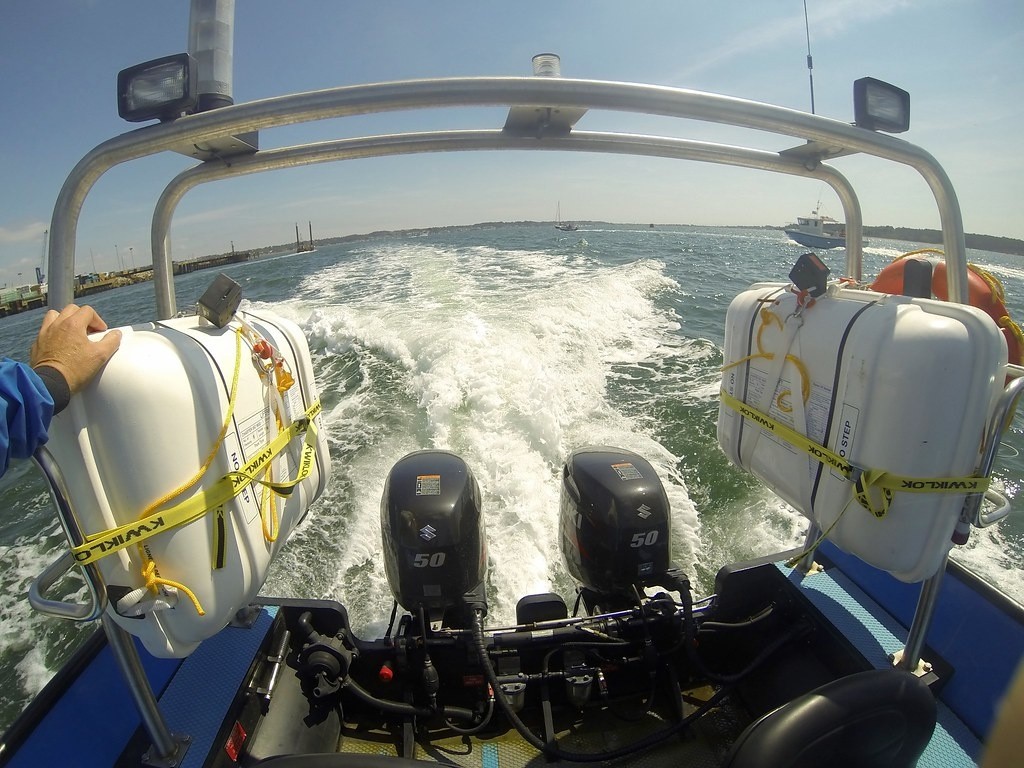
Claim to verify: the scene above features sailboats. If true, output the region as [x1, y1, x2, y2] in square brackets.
[295, 220, 314, 253]
[555, 200, 578, 230]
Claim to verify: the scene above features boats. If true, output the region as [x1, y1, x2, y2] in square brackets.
[785, 200, 869, 249]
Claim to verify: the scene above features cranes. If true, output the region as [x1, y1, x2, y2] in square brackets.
[34, 229, 48, 284]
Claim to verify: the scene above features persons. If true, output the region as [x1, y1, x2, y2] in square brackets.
[0, 304, 121, 482]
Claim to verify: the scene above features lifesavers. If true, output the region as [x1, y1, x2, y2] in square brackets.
[871, 250, 1024, 433]
